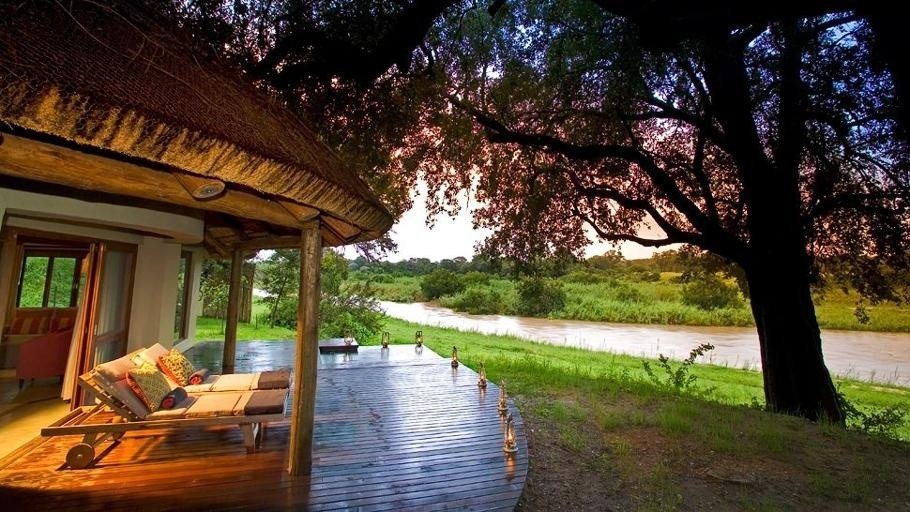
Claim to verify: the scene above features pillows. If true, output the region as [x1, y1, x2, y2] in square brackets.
[158, 345, 197, 387]
[126, 360, 171, 413]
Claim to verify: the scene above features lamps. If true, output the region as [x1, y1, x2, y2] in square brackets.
[503, 452, 516, 483]
[497, 380, 509, 411]
[479, 387, 486, 405]
[503, 414, 518, 453]
[498, 411, 508, 433]
[451, 346, 458, 367]
[381, 331, 389, 348]
[477, 362, 487, 387]
[415, 331, 423, 347]
[344, 329, 353, 346]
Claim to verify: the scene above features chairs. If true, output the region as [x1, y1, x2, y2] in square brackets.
[95, 343, 295, 441]
[16, 327, 73, 389]
[0, 335, 32, 369]
[40, 352, 289, 469]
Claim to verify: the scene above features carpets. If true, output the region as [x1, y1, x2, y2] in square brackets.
[0, 367, 62, 417]
